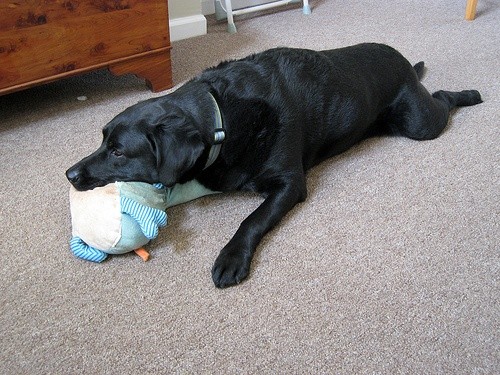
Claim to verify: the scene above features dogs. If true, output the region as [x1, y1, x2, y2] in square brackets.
[65, 43, 484, 290]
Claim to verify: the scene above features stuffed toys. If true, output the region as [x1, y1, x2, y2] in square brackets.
[66, 181, 212, 264]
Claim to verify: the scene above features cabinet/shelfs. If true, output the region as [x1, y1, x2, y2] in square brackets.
[0, 0, 173, 95]
